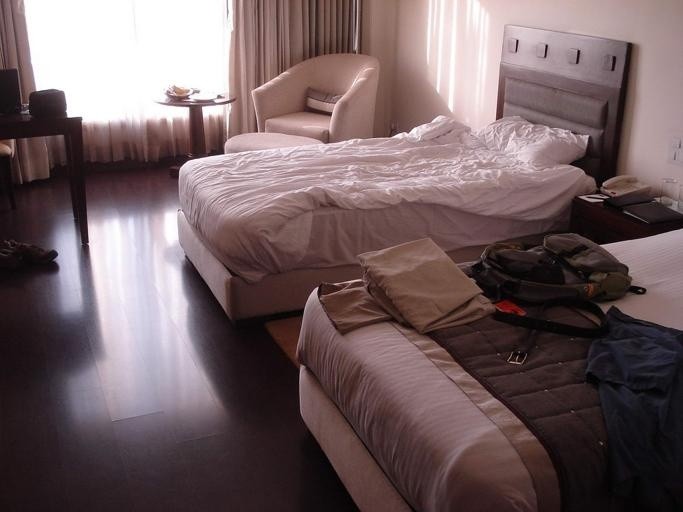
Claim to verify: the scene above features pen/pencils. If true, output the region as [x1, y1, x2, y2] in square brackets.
[587, 197, 604, 199]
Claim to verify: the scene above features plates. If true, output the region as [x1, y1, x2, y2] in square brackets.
[194, 96, 217, 101]
[166, 88, 193, 101]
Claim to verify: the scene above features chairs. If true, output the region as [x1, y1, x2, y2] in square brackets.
[0, 139, 21, 210]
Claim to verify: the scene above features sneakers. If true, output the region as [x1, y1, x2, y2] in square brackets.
[0, 238, 57, 265]
[0, 247, 23, 272]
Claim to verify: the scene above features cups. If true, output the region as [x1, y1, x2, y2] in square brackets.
[678, 185, 683, 210]
[660, 177, 677, 208]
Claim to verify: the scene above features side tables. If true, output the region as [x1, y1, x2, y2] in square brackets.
[154, 95, 237, 177]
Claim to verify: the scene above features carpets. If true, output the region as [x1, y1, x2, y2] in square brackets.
[265, 317, 301, 368]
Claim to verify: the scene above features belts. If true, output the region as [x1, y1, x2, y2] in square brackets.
[491, 297, 610, 364]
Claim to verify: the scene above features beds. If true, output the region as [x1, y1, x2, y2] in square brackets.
[177, 25, 631, 326]
[296, 227, 683, 512]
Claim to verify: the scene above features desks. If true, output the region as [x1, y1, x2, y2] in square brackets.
[0, 109, 89, 245]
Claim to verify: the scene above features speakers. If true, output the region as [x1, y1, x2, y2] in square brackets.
[0, 68, 21, 113]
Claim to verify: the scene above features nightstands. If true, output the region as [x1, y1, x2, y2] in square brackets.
[571, 193, 683, 246]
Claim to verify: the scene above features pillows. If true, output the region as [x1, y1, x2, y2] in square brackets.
[304, 87, 345, 116]
[471, 116, 589, 172]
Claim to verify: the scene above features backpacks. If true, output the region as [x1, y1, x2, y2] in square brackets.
[470, 233, 632, 303]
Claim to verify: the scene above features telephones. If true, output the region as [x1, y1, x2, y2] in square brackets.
[600, 175, 652, 206]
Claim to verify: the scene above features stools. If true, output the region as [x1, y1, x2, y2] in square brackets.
[224, 132, 323, 154]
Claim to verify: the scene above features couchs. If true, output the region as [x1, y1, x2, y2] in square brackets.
[251, 54, 380, 144]
